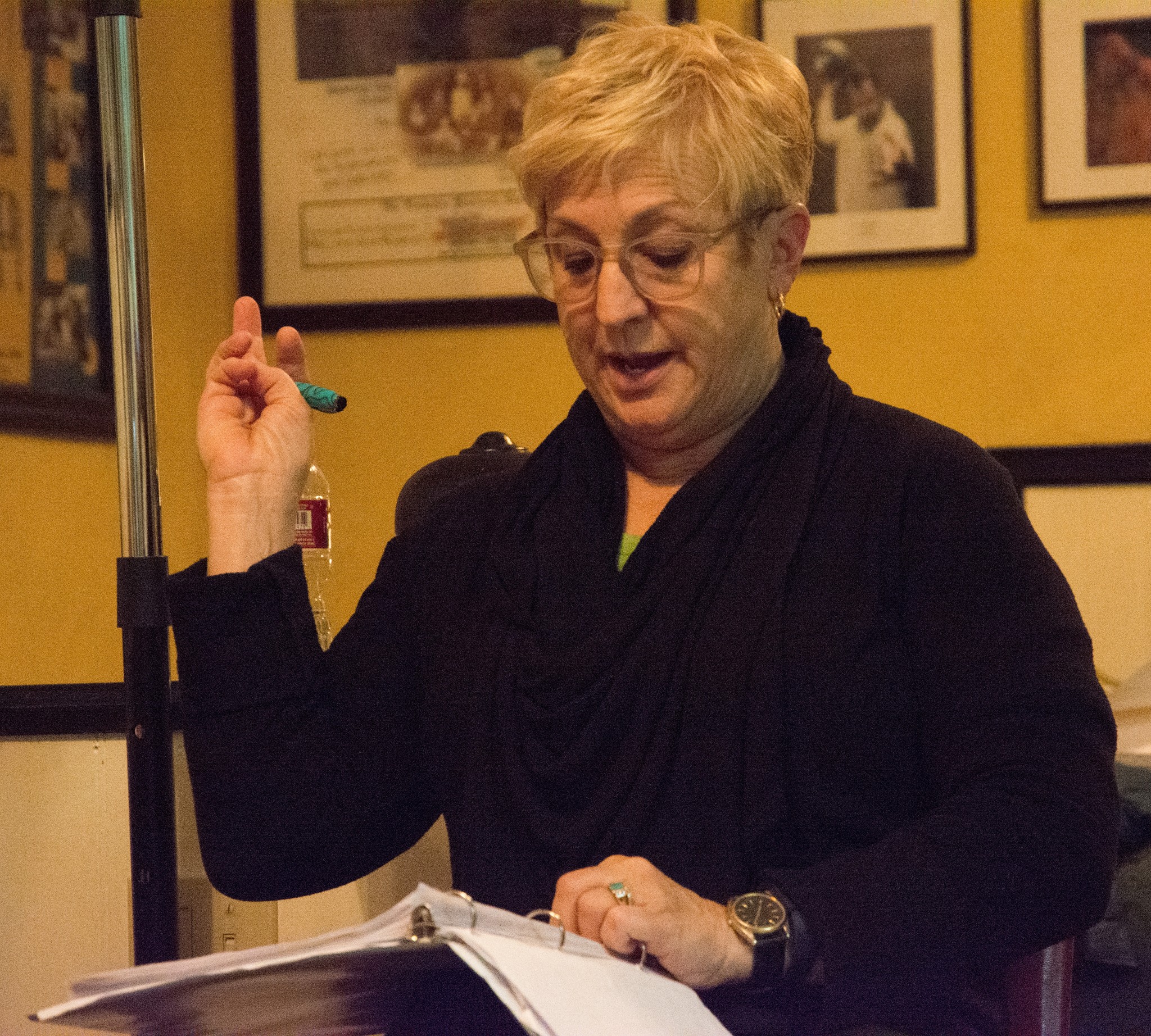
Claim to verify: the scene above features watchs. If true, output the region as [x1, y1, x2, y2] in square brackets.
[724, 890, 789, 987]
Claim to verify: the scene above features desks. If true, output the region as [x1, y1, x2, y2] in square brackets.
[0, 944, 1009, 1036]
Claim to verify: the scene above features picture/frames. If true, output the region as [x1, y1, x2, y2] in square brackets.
[229, 0, 700, 334]
[1032, 0, 1151, 212]
[755, 0, 977, 267]
[0, 0, 121, 447]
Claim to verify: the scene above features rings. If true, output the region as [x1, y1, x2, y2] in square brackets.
[608, 882, 628, 906]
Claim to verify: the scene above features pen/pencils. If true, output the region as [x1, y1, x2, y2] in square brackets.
[297, 380, 348, 413]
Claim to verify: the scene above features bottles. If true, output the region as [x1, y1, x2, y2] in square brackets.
[460, 429, 525, 452]
[294, 459, 333, 656]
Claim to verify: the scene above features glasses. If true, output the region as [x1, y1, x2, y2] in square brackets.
[511, 199, 788, 304]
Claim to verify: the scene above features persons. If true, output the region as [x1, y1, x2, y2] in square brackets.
[161, 16, 1123, 1036]
[811, 64, 916, 212]
